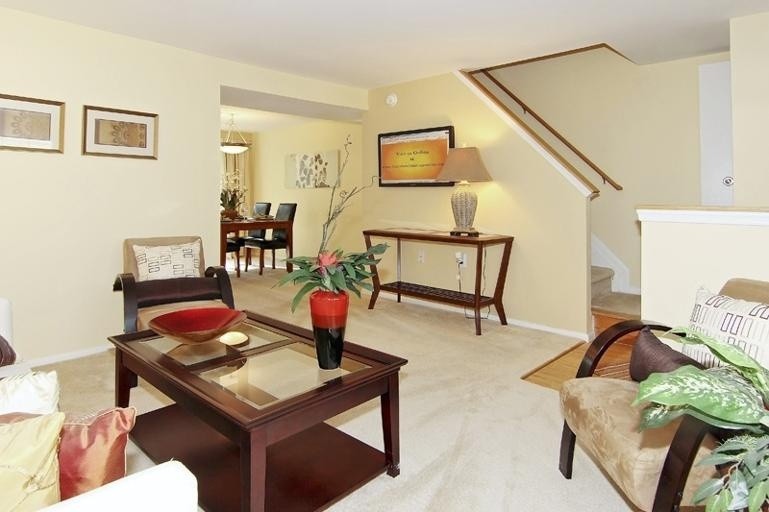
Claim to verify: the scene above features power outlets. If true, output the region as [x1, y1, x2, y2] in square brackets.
[456, 254, 467, 268]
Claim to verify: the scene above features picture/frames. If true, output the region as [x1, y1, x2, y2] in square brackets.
[378, 125, 455, 188]
[0, 94, 159, 160]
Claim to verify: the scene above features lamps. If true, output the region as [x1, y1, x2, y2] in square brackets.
[436, 147, 492, 237]
[220, 113, 252, 154]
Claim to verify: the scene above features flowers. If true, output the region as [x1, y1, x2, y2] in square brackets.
[267, 243, 389, 315]
[220, 169, 248, 210]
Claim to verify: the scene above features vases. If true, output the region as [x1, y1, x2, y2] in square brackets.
[310, 289, 350, 370]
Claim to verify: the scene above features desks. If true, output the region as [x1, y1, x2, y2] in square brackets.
[362, 226, 514, 335]
[220, 217, 293, 273]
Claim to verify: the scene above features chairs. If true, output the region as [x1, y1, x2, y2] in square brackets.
[226, 238, 241, 278]
[113, 236, 235, 334]
[558, 277, 769, 512]
[225, 202, 297, 278]
[0, 298, 199, 512]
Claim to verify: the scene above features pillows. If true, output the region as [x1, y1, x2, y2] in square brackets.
[0, 359, 138, 512]
[132, 240, 200, 283]
[629, 287, 768, 443]
